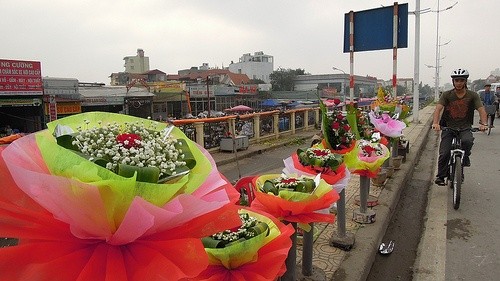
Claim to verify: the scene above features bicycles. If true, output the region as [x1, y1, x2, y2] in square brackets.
[430, 125, 488, 210]
[480, 101, 497, 136]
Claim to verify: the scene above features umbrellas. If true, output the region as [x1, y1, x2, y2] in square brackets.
[277, 100, 318, 109]
[229, 104, 253, 112]
[259, 99, 278, 107]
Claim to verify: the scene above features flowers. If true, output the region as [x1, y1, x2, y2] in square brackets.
[52, 113, 197, 184]
[199, 211, 271, 249]
[257, 85, 412, 203]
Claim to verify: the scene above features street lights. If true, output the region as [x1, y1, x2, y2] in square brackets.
[380, 0, 458, 124]
[331, 66, 347, 102]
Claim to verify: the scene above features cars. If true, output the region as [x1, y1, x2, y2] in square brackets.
[478, 82, 500, 105]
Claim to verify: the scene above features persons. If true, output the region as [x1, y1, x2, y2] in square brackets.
[479, 84, 500, 128]
[432, 68, 486, 187]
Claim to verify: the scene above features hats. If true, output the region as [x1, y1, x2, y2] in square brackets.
[484, 84, 491, 87]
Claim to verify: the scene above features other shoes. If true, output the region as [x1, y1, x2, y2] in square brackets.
[435, 178, 445, 184]
[463, 156, 470, 166]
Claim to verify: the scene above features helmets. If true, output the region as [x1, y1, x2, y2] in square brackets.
[451, 68, 469, 78]
